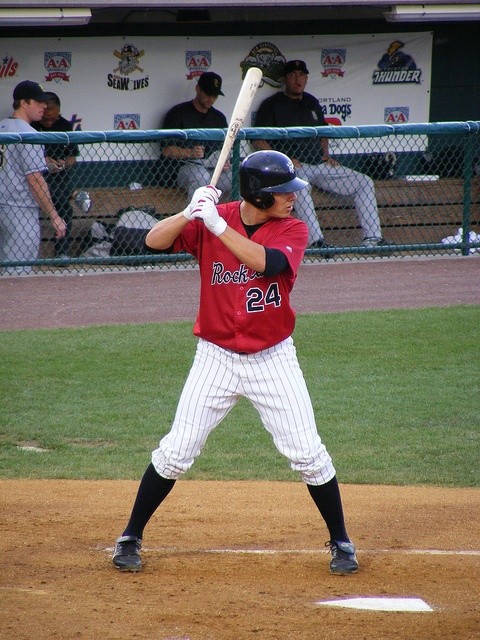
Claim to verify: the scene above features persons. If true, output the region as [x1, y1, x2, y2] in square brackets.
[0, 80, 66, 268]
[160, 73, 243, 204]
[112, 150, 359, 576]
[253, 59, 387, 249]
[31, 92, 78, 257]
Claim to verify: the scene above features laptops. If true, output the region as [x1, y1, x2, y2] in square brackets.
[183, 145, 234, 168]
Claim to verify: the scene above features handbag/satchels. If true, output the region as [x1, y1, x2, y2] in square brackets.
[368, 149, 397, 180]
[82, 221, 114, 256]
[110, 210, 159, 254]
[421, 145, 480, 179]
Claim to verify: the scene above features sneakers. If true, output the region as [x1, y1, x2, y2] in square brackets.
[310, 240, 336, 259]
[74, 191, 90, 213]
[361, 236, 397, 255]
[112, 535, 142, 572]
[329, 539, 358, 575]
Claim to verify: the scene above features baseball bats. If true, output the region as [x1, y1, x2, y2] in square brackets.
[210, 67, 263, 187]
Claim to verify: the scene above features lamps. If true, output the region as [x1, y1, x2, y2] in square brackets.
[0, 7, 93, 27]
[382, 3, 480, 22]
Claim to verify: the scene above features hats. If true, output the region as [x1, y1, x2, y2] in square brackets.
[284, 60, 309, 74]
[199, 72, 225, 96]
[13, 80, 56, 100]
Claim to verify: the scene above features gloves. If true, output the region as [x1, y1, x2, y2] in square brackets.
[190, 197, 228, 236]
[183, 185, 222, 221]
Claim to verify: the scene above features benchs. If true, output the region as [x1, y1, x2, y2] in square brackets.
[39, 177, 480, 261]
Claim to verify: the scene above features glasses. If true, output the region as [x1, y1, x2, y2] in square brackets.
[42, 116, 59, 121]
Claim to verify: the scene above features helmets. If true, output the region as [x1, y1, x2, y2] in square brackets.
[240, 150, 309, 209]
